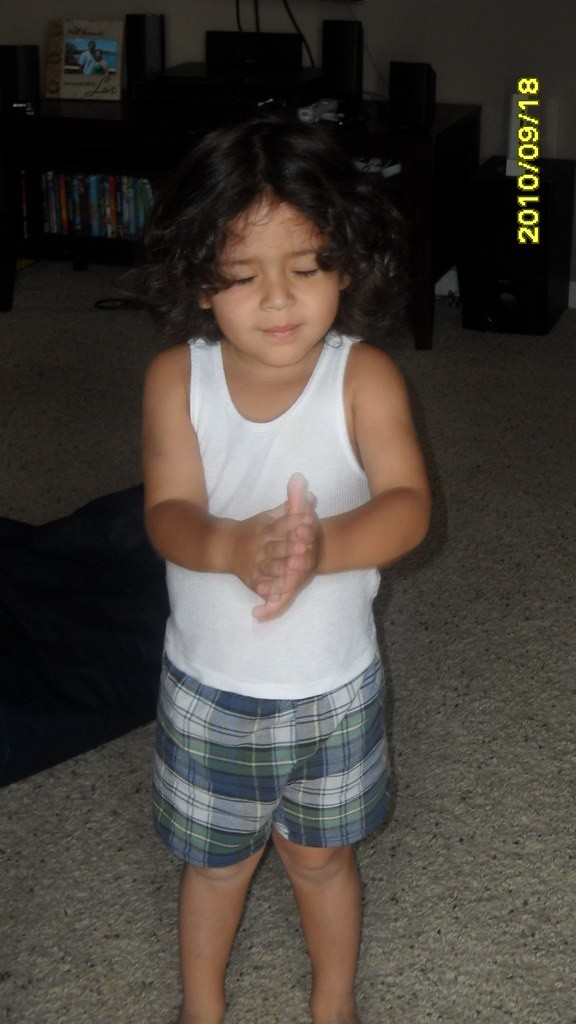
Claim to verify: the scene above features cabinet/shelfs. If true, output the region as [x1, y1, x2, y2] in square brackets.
[19, 119, 174, 264]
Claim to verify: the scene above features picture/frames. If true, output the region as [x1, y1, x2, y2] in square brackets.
[45, 17, 123, 101]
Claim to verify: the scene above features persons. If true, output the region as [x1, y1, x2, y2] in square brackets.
[121, 111, 431, 1024]
[78, 41, 108, 75]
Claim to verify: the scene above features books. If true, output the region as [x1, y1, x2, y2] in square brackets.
[44, 16, 124, 101]
[21, 170, 166, 238]
